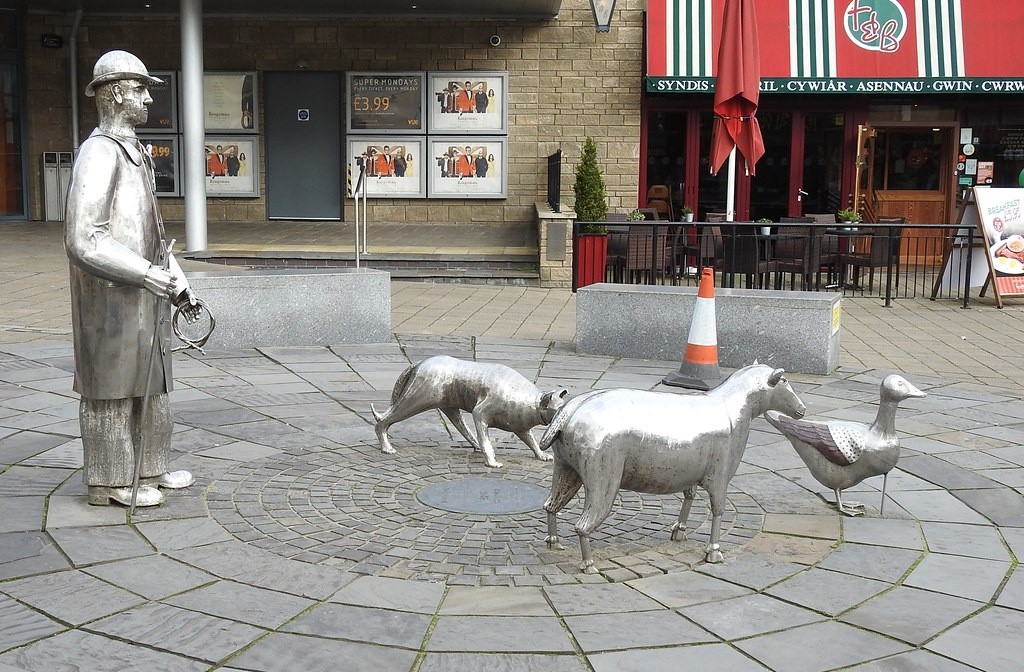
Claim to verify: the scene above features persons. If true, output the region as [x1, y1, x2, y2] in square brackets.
[205, 145, 247, 177]
[63, 50, 203, 507]
[437, 146, 495, 178]
[242, 75, 253, 129]
[362, 145, 414, 177]
[437, 81, 495, 113]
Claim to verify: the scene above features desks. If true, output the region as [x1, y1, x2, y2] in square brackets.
[607, 229, 629, 284]
[820, 227, 874, 289]
[758, 234, 810, 289]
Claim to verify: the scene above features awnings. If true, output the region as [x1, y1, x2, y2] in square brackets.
[646, 0, 1024, 94]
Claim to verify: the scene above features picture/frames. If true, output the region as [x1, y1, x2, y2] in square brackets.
[135, 72, 261, 199]
[346, 70, 509, 200]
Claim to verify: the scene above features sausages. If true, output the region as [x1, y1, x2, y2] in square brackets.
[995, 243, 1007, 258]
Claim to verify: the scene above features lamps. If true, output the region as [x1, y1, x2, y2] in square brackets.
[590, 0, 616, 33]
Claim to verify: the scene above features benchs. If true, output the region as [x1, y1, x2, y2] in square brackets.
[575, 282, 841, 374]
[172, 268, 392, 353]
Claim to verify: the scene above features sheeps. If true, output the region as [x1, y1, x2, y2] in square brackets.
[540, 358, 806, 576]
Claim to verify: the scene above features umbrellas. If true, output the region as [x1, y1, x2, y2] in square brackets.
[710, 0, 765, 177]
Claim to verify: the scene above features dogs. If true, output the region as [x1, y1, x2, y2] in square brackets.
[371, 354, 567, 467]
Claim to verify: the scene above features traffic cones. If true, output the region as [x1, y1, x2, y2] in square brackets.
[662, 268, 731, 390]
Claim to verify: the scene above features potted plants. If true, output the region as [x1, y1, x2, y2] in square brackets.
[838, 210, 863, 232]
[682, 205, 694, 223]
[572, 135, 609, 288]
[758, 218, 773, 235]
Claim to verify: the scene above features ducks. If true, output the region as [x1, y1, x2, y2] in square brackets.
[764, 374, 927, 517]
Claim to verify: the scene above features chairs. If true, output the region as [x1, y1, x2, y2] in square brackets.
[605, 185, 906, 297]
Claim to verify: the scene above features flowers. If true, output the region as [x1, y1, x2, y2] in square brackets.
[626, 209, 645, 221]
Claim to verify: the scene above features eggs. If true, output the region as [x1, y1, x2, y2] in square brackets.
[993, 256, 1024, 273]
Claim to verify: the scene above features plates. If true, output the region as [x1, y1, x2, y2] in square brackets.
[990, 239, 1024, 275]
[1006, 235, 1024, 253]
